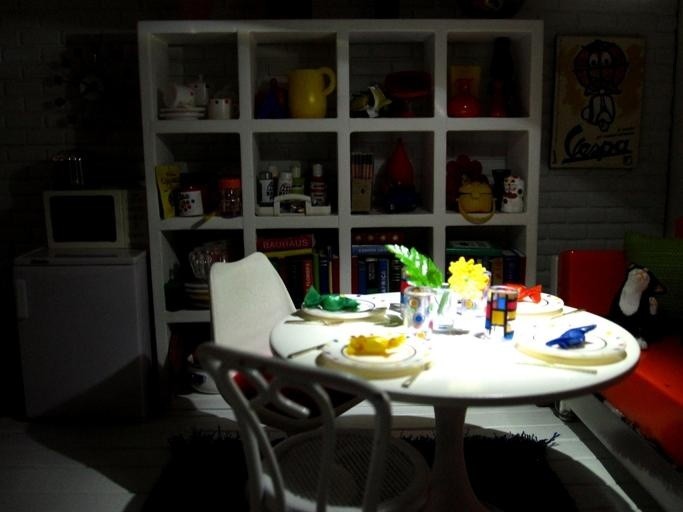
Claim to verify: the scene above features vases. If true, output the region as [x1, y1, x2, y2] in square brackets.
[429, 284, 458, 333]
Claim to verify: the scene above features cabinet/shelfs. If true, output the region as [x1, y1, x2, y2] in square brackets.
[138, 18, 545, 371]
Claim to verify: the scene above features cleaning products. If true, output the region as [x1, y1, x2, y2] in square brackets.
[257, 164, 324, 206]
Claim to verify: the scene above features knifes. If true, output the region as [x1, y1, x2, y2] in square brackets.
[288, 340, 339, 360]
[284, 316, 341, 326]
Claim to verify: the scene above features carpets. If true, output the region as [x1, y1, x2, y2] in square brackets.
[139, 425, 578, 512]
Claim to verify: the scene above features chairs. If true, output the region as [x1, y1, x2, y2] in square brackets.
[198, 342, 431, 512]
[208, 251, 365, 433]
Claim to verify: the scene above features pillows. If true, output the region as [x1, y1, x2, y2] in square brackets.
[624, 230, 683, 335]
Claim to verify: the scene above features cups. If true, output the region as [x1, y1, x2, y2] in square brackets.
[404, 288, 455, 338]
[160, 76, 232, 119]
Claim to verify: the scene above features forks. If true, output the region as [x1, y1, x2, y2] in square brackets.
[402, 350, 433, 387]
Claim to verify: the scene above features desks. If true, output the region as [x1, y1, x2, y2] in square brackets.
[270, 292, 641, 512]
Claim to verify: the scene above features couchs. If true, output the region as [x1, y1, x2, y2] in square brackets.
[559, 217, 683, 474]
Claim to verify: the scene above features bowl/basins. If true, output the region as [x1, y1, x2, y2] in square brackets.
[182, 247, 227, 309]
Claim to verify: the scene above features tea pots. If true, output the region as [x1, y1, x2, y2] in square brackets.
[284, 67, 336, 118]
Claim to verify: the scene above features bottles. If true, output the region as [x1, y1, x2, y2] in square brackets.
[177, 170, 213, 221]
[217, 177, 242, 218]
[258, 164, 329, 210]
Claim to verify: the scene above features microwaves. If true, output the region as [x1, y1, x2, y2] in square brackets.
[40, 188, 134, 252]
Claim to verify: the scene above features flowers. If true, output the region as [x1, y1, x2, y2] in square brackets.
[384, 244, 490, 300]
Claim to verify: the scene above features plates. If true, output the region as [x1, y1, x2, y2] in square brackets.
[304, 296, 375, 320]
[515, 291, 565, 314]
[521, 330, 626, 364]
[323, 337, 424, 372]
